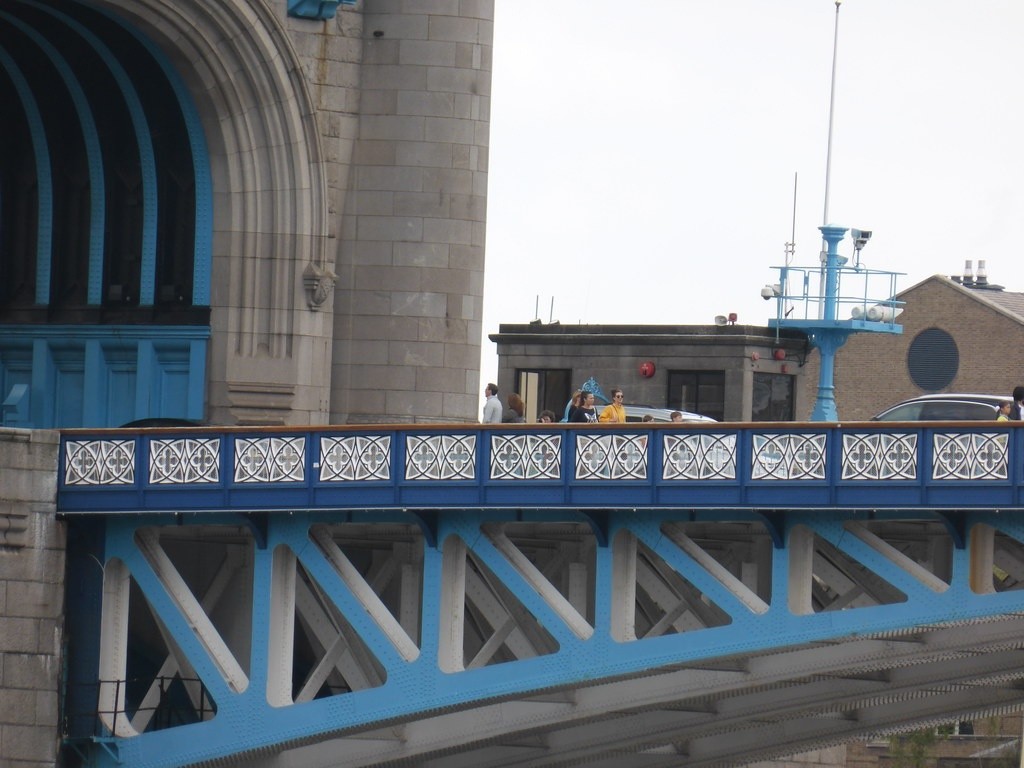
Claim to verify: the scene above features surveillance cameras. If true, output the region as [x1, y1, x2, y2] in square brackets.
[851, 228, 872, 241]
[761, 287, 774, 300]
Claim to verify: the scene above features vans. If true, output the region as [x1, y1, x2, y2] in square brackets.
[596, 405, 718, 423]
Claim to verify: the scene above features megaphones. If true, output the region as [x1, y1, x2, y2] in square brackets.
[715, 316, 727, 326]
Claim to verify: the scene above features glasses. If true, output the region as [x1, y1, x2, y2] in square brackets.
[616, 395, 624, 398]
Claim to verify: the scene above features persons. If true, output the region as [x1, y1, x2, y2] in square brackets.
[482, 384, 503, 476]
[536, 388, 682, 473]
[996, 385, 1024, 475]
[502, 392, 525, 459]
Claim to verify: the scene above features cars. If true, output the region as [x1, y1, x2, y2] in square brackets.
[861, 394, 1018, 420]
